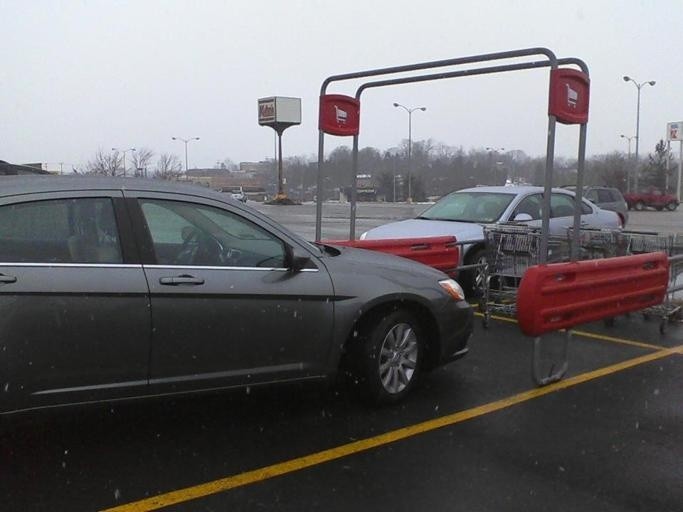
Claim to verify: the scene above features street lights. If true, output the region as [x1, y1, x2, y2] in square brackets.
[112, 147, 136, 176]
[44, 160, 48, 172]
[393, 102, 426, 203]
[59, 162, 65, 175]
[620, 134, 636, 193]
[486, 146, 505, 186]
[171, 136, 200, 175]
[623, 76, 656, 193]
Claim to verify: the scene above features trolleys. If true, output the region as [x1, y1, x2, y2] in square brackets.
[481, 220, 683, 335]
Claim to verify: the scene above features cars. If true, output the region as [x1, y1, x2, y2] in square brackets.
[0, 160, 50, 176]
[359, 186, 623, 298]
[1, 176, 474, 409]
[231, 189, 248, 203]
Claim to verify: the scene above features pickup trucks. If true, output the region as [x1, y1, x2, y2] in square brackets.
[623, 187, 680, 212]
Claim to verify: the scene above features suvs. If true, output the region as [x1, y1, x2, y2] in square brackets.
[561, 187, 630, 230]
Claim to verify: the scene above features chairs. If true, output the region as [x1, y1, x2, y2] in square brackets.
[69, 218, 93, 265]
[555, 205, 573, 217]
[94, 203, 121, 264]
[481, 201, 501, 221]
